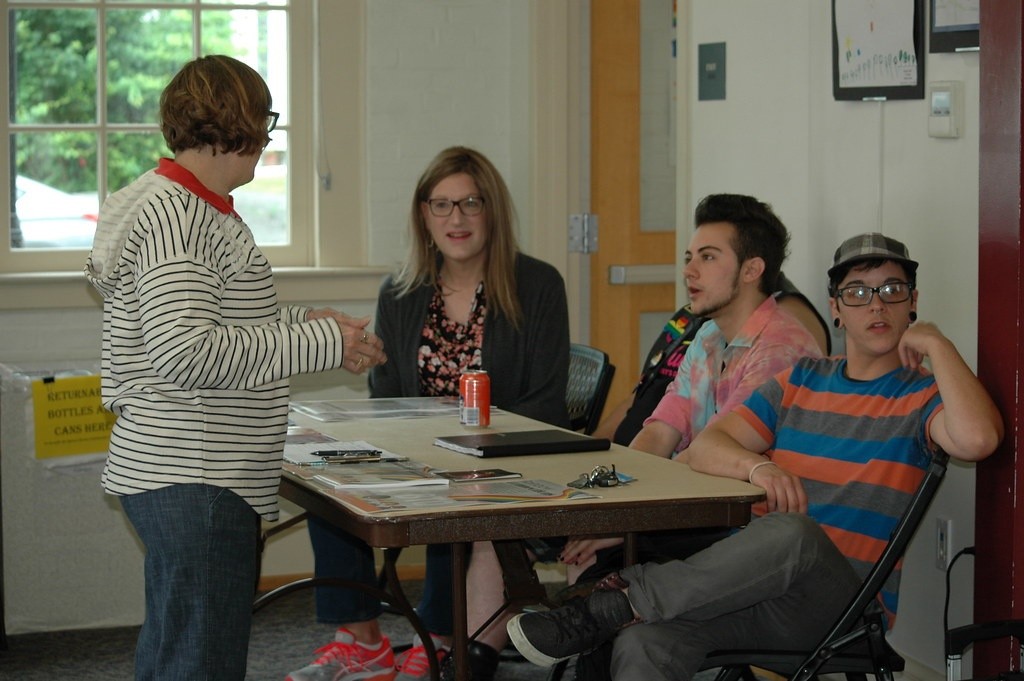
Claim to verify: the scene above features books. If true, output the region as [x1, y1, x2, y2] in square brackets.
[313, 462, 449, 489]
[432, 469, 522, 482]
[433, 429, 610, 458]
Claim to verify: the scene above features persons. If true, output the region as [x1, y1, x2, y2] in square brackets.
[428, 274, 831, 681]
[448, 234, 1006, 681]
[555, 195, 824, 681]
[84, 54, 388, 681]
[285, 147, 570, 680]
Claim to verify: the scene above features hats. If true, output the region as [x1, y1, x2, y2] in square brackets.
[828, 232, 919, 278]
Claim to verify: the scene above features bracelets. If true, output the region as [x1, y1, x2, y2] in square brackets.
[749, 462, 775, 483]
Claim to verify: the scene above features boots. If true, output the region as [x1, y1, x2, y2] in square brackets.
[439, 638, 500, 681]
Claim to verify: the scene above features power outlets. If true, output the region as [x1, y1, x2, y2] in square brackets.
[937, 519, 952, 569]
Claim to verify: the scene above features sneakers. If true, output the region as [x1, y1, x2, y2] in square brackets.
[507, 573, 640, 669]
[395, 632, 453, 681]
[285, 628, 399, 681]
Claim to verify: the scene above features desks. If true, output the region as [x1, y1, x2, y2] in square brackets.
[252, 398, 767, 680]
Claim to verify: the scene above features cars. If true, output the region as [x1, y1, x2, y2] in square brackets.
[9, 174, 111, 251]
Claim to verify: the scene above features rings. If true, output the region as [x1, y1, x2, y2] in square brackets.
[360, 333, 368, 342]
[357, 354, 364, 367]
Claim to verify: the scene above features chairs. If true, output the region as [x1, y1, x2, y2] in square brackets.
[368, 341, 954, 680]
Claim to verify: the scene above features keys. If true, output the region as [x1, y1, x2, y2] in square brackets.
[595, 471, 619, 487]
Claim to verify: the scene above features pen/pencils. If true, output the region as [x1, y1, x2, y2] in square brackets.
[310, 450, 382, 457]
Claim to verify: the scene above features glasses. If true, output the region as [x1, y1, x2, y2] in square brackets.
[266, 111, 279, 132]
[424, 197, 485, 217]
[835, 282, 912, 306]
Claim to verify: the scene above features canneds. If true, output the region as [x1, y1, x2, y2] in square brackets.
[459, 369, 491, 428]
